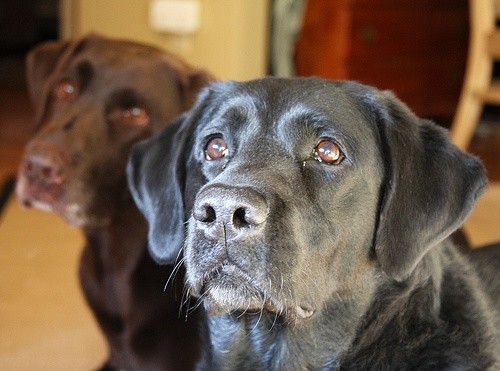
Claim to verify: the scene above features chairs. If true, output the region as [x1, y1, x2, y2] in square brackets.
[449, 1, 500, 153]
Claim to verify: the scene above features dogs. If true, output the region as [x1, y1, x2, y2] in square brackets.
[124, 77, 500, 371]
[17, 32, 208, 371]
